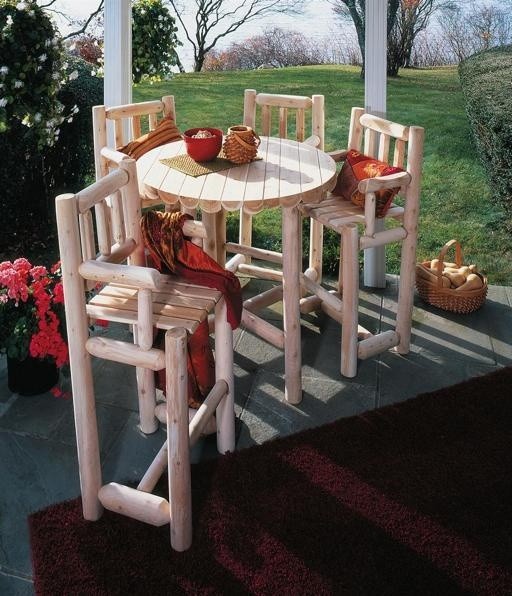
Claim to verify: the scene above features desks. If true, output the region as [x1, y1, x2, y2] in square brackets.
[135, 134, 337, 405]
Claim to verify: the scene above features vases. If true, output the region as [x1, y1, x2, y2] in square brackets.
[6, 353, 58, 396]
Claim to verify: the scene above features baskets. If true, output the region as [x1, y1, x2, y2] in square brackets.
[414, 239, 487, 314]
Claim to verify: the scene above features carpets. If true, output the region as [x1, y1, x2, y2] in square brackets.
[29, 363, 512, 594]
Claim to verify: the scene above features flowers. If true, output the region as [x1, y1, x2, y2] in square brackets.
[0, 258, 110, 379]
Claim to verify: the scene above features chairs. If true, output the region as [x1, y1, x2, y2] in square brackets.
[91, 90, 326, 300]
[297, 106, 425, 378]
[55, 157, 235, 552]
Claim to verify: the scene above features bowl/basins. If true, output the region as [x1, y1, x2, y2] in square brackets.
[181, 127, 223, 163]
